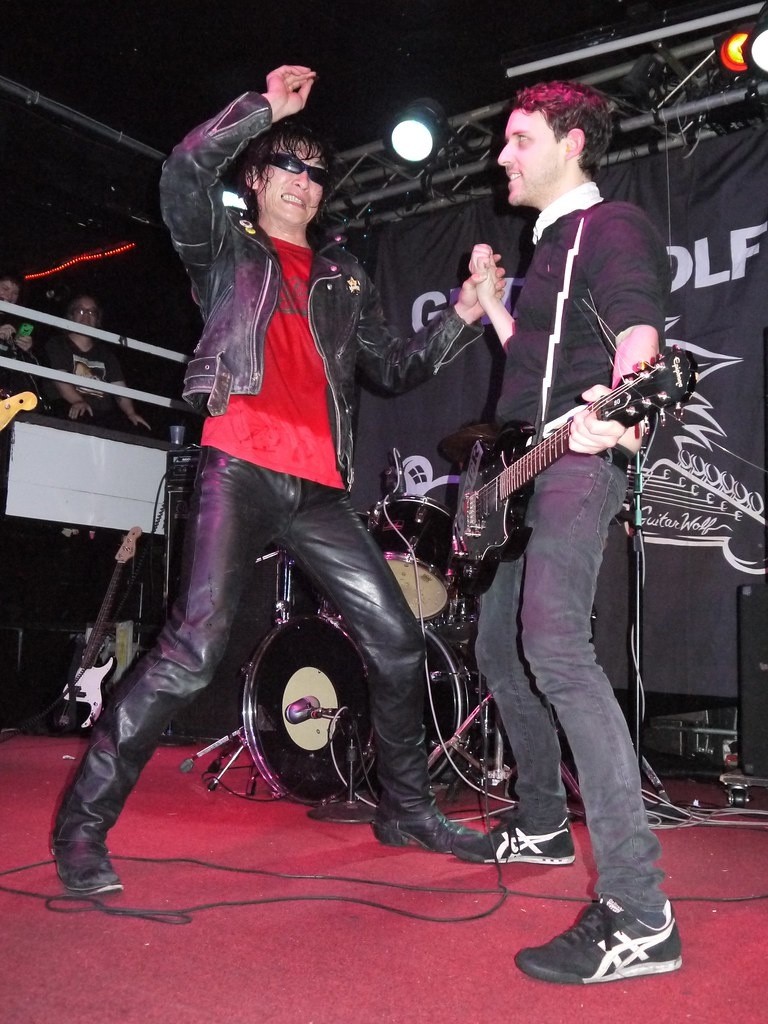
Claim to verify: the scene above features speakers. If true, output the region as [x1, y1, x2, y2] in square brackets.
[740, 585, 767, 779]
[162, 485, 321, 749]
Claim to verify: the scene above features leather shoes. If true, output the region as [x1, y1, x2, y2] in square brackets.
[51, 839, 124, 896]
[373, 811, 485, 854]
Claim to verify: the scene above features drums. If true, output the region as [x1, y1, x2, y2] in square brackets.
[241, 613, 470, 808]
[280, 511, 372, 624]
[368, 493, 457, 622]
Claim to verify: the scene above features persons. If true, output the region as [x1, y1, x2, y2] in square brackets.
[0, 271, 153, 437]
[54, 64, 506, 897]
[469, 80, 683, 985]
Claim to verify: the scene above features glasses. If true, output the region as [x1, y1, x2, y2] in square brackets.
[72, 304, 99, 316]
[267, 153, 328, 187]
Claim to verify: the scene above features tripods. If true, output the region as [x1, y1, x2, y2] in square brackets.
[427, 691, 586, 797]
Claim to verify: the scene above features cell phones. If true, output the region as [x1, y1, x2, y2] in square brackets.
[15, 323, 34, 339]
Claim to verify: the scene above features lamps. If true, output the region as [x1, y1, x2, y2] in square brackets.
[620, 53, 666, 103]
[384, 96, 450, 168]
[713, 2, 768, 83]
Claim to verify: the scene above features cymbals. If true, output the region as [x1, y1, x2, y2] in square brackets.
[441, 423, 497, 461]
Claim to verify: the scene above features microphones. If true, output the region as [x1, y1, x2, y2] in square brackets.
[397, 457, 406, 493]
[285, 696, 321, 724]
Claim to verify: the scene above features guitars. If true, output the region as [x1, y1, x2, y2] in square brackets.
[0, 390, 40, 432]
[52, 525, 143, 731]
[450, 343, 700, 601]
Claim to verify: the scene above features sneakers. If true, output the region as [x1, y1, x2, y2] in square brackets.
[452, 820, 576, 866]
[514, 896, 683, 985]
